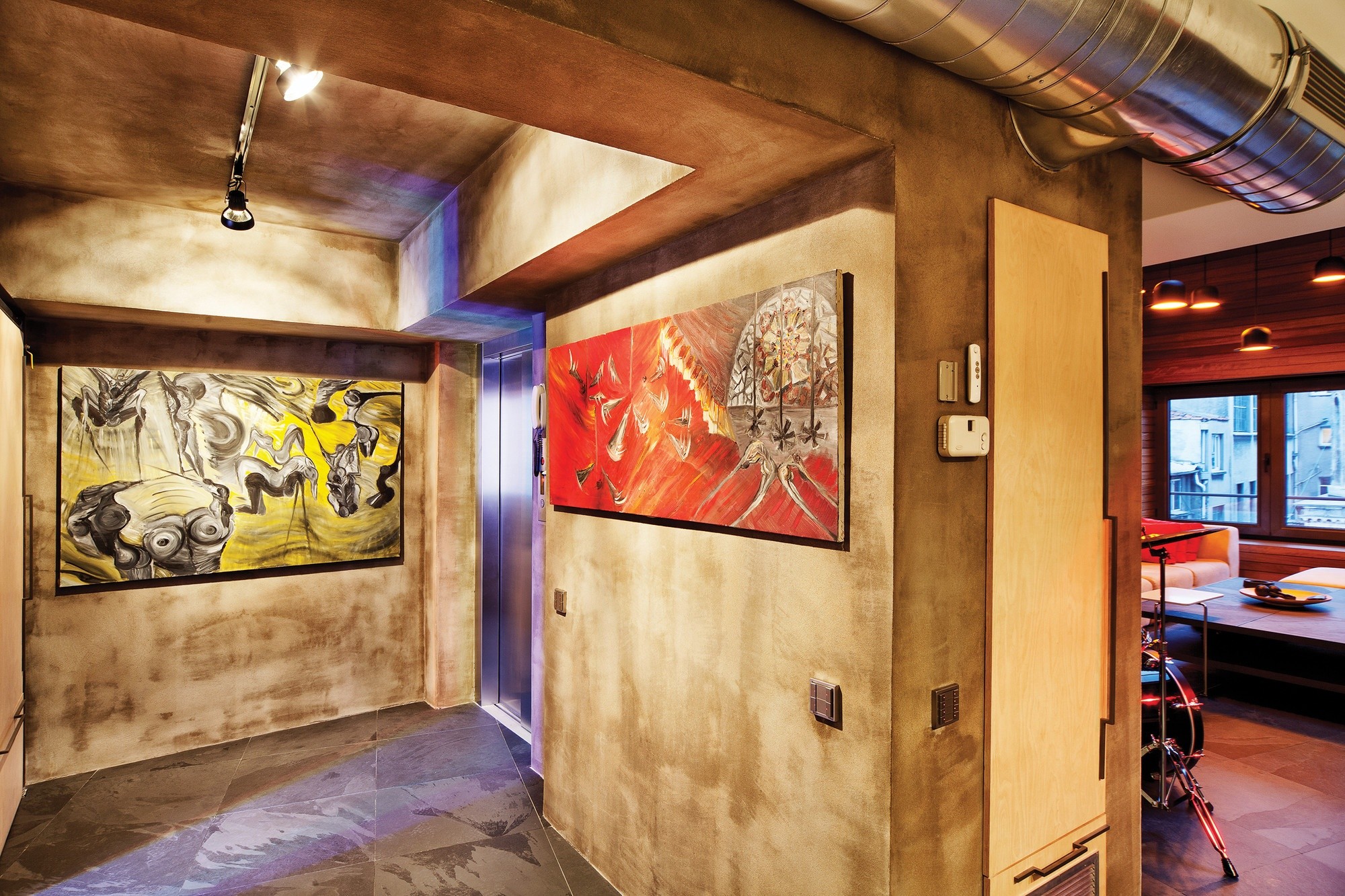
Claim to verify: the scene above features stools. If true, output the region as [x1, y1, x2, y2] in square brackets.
[1280, 567, 1345, 591]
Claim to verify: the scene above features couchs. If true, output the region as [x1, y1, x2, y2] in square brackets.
[1137, 515, 1240, 634]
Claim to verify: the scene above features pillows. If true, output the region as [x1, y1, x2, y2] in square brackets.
[1141, 518, 1205, 565]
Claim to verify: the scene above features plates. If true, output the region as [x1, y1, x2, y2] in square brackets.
[1239, 588, 1332, 608]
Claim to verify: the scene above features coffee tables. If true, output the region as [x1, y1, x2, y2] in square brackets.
[1136, 576, 1345, 704]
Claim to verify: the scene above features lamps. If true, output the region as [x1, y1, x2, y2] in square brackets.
[218, 52, 325, 232]
[1142, 232, 1345, 355]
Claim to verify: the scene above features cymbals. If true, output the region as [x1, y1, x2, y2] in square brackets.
[1142, 524, 1230, 548]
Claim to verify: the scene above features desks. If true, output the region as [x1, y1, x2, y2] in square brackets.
[1140, 585, 1224, 699]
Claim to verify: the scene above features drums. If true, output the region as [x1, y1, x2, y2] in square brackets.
[1142, 646, 1204, 778]
